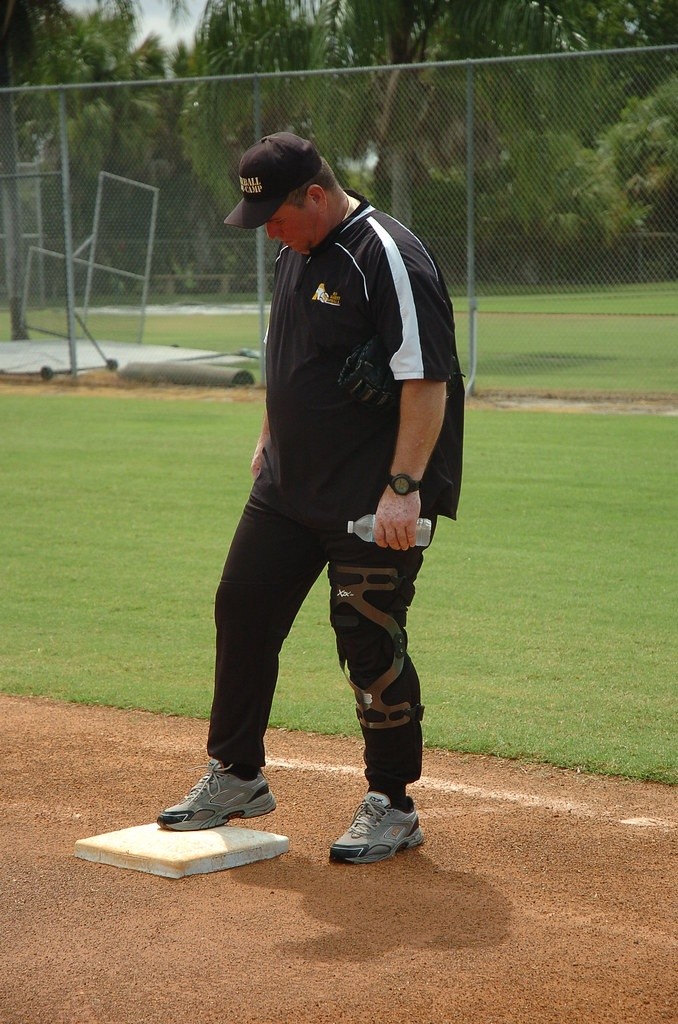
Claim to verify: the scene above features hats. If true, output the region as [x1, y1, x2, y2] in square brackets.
[223, 131, 322, 229]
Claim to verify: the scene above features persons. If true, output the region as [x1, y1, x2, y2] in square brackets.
[154, 127, 466, 865]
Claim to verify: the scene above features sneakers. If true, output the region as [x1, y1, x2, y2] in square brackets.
[330, 791, 424, 864]
[157, 759, 276, 832]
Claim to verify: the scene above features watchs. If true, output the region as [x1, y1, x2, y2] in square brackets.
[386, 472, 423, 496]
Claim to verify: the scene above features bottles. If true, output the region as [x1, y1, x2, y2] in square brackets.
[348, 514, 432, 546]
